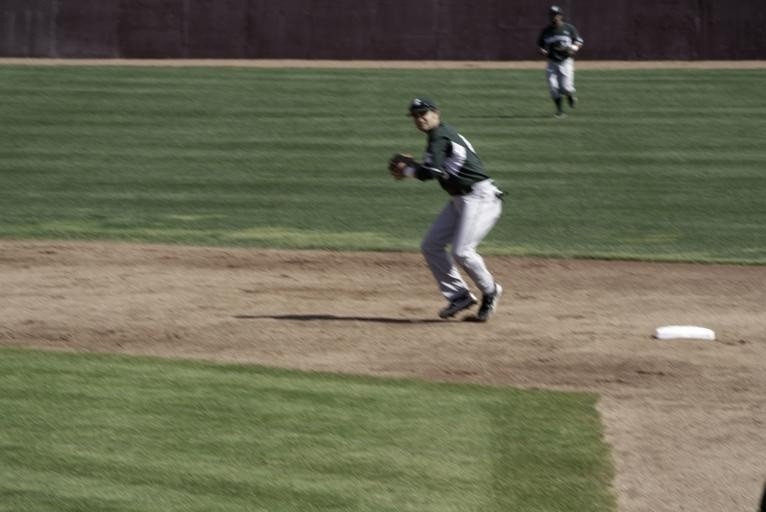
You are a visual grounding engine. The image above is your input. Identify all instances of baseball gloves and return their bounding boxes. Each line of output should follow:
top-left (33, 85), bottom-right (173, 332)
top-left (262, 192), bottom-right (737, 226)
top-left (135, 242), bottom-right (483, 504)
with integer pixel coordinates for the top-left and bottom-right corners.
top-left (548, 46), bottom-right (569, 62)
top-left (389, 153), bottom-right (413, 179)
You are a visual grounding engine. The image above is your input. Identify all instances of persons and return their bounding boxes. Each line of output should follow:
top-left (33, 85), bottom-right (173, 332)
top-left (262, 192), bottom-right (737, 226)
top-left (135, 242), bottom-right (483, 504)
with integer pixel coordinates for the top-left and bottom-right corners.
top-left (386, 96), bottom-right (508, 323)
top-left (535, 3), bottom-right (584, 118)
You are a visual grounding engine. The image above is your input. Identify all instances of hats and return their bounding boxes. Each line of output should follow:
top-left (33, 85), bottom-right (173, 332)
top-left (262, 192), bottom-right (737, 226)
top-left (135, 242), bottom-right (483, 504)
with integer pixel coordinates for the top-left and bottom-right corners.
top-left (407, 96), bottom-right (437, 117)
top-left (549, 5), bottom-right (564, 15)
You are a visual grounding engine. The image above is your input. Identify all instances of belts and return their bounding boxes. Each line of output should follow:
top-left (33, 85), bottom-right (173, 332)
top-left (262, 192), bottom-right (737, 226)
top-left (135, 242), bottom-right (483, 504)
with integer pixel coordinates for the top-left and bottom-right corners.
top-left (450, 187), bottom-right (472, 196)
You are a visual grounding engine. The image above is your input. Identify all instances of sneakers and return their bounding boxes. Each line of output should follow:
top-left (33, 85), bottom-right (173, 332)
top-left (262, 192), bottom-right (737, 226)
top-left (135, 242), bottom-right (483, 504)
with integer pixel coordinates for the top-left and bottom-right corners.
top-left (568, 95), bottom-right (575, 107)
top-left (479, 283), bottom-right (502, 321)
top-left (555, 109), bottom-right (564, 117)
top-left (440, 292), bottom-right (477, 318)
